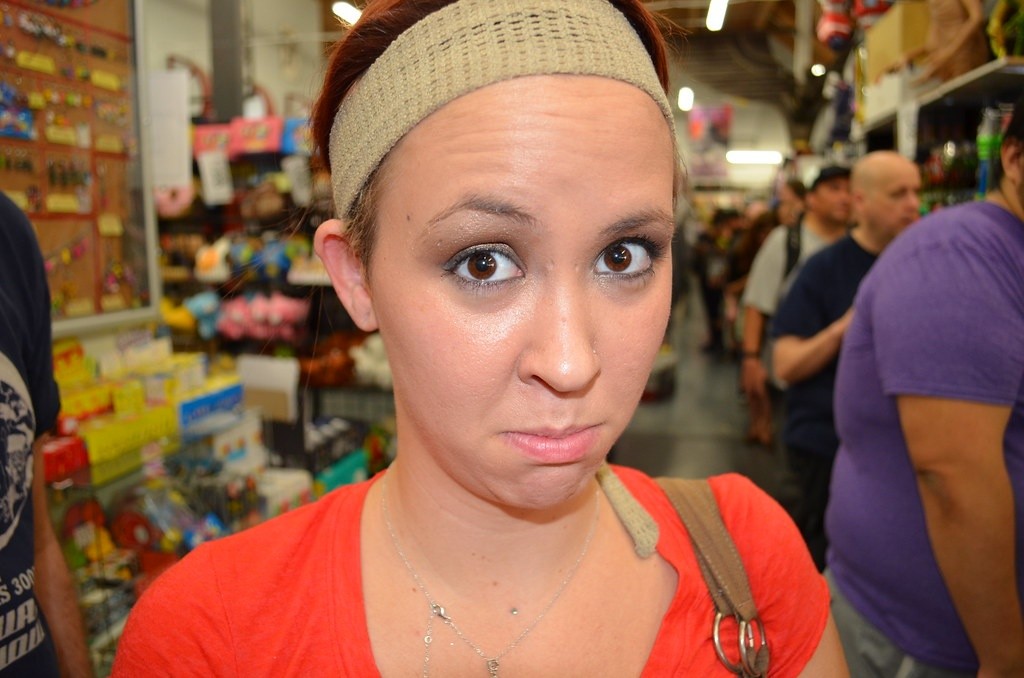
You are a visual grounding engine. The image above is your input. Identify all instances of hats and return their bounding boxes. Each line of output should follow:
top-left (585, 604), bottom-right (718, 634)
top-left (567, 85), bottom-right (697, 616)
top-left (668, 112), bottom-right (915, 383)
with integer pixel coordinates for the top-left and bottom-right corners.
top-left (799, 161), bottom-right (850, 193)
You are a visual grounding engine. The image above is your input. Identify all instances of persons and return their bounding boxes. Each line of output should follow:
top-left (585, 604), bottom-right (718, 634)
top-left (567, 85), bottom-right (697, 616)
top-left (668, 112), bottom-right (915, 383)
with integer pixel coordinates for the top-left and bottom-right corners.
top-left (763, 146), bottom-right (931, 588)
top-left (738, 163), bottom-right (857, 516)
top-left (0, 188), bottom-right (100, 678)
top-left (663, 173), bottom-right (807, 449)
top-left (97, 0), bottom-right (853, 678)
top-left (806, 87), bottom-right (1024, 678)
